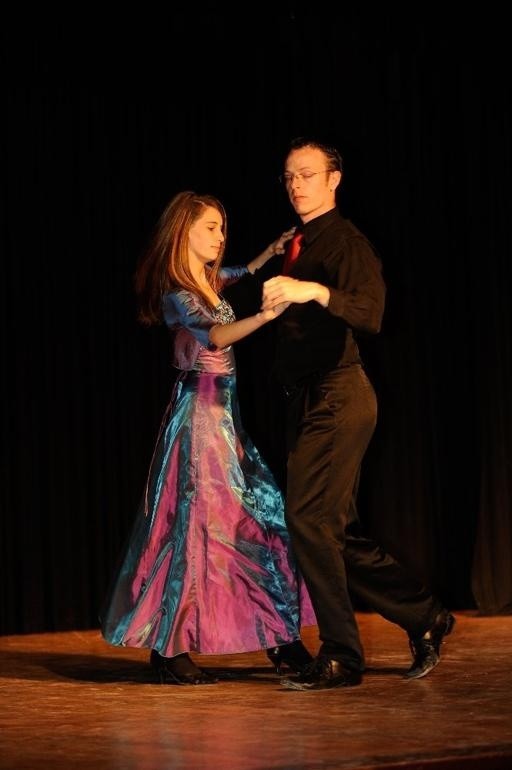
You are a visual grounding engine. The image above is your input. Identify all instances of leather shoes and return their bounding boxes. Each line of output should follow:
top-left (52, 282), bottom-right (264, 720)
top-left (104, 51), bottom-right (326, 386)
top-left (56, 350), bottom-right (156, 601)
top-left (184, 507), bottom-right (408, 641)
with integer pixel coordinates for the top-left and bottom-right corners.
top-left (402, 606), bottom-right (458, 681)
top-left (277, 653), bottom-right (366, 692)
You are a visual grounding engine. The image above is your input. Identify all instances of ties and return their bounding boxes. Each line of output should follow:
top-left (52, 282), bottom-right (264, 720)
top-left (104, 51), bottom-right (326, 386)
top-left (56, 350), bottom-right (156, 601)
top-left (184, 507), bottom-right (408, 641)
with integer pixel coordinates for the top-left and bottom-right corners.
top-left (282, 233), bottom-right (305, 275)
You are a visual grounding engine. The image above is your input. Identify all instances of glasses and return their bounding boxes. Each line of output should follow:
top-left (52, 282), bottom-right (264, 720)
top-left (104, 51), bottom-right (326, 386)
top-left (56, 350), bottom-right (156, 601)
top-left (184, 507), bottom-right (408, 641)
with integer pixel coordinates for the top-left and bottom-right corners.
top-left (279, 168), bottom-right (332, 187)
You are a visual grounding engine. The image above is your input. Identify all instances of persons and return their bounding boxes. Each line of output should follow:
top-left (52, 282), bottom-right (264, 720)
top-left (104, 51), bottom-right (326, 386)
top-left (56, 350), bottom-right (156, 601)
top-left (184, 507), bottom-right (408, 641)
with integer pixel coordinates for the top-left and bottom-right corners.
top-left (101, 190), bottom-right (319, 686)
top-left (261, 136), bottom-right (454, 692)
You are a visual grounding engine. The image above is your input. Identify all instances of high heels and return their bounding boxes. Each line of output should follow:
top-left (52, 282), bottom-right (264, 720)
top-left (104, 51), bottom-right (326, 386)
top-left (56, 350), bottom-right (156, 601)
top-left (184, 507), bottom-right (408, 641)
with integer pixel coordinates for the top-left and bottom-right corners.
top-left (150, 649), bottom-right (220, 686)
top-left (266, 640), bottom-right (316, 674)
